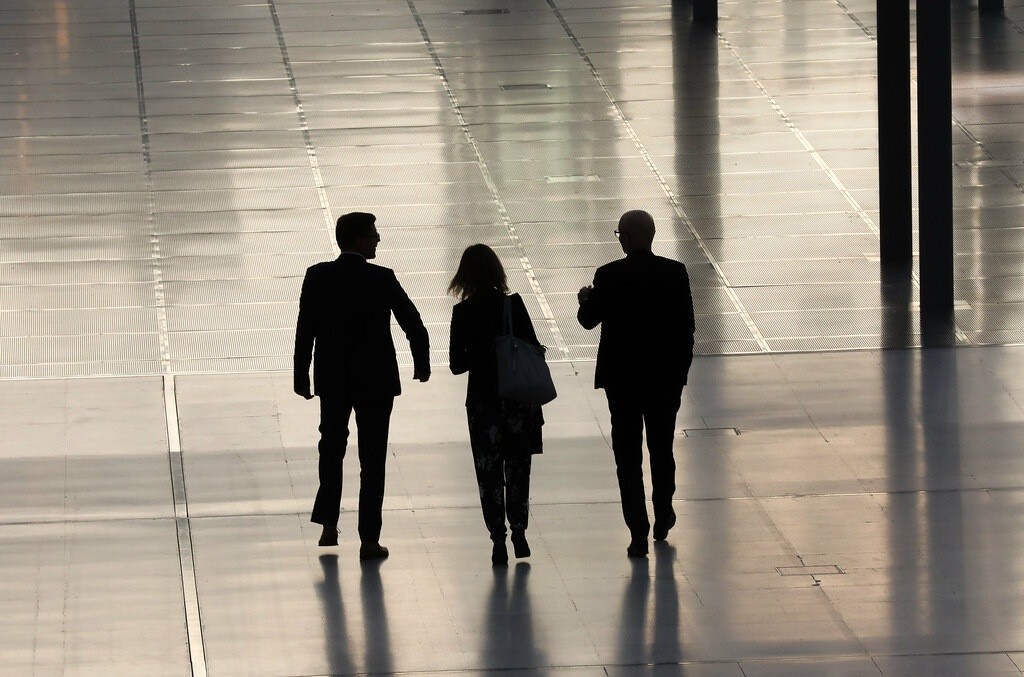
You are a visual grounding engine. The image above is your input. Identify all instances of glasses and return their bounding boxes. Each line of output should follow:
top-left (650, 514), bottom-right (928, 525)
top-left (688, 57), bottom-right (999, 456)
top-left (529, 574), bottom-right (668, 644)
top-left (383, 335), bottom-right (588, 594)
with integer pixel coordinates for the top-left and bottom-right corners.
top-left (614, 229), bottom-right (621, 238)
top-left (357, 233), bottom-right (379, 240)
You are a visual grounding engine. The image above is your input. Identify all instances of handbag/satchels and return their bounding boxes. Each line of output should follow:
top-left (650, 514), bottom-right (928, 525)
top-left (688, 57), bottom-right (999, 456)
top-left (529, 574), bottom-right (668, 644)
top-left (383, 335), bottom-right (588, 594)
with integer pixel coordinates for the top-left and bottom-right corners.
top-left (494, 296), bottom-right (557, 406)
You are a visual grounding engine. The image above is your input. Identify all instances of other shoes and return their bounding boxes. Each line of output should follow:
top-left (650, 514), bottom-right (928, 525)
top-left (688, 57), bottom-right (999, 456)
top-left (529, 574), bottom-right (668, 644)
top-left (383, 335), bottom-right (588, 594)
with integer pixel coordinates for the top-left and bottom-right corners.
top-left (652, 510), bottom-right (676, 540)
top-left (360, 543), bottom-right (389, 560)
top-left (511, 530), bottom-right (530, 558)
top-left (492, 547), bottom-right (508, 565)
top-left (627, 539), bottom-right (648, 555)
top-left (318, 525), bottom-right (341, 546)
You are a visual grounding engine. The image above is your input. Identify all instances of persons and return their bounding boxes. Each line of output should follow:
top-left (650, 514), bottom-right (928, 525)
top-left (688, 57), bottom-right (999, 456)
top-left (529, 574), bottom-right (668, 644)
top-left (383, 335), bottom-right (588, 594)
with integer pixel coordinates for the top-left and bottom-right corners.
top-left (577, 209), bottom-right (696, 557)
top-left (447, 243), bottom-right (544, 565)
top-left (293, 212), bottom-right (430, 561)
